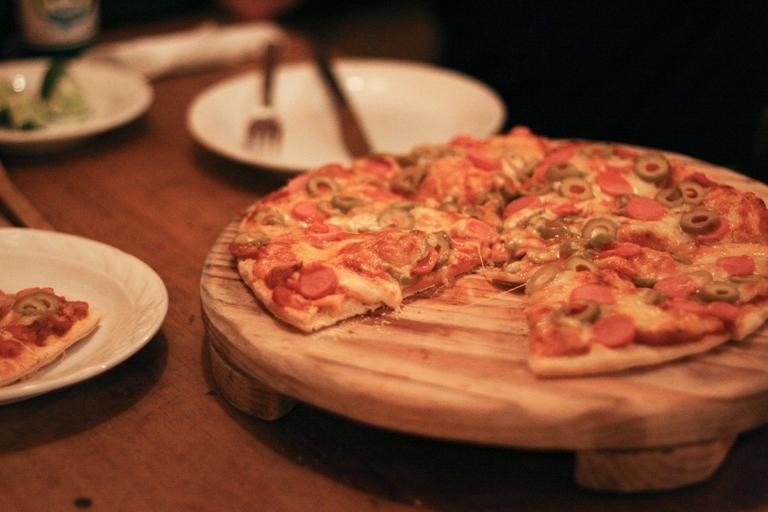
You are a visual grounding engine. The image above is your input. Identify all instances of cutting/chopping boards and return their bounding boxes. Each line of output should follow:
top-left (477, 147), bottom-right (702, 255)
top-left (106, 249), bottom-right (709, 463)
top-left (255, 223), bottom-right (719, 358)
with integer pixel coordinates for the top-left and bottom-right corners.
top-left (197, 138), bottom-right (768, 493)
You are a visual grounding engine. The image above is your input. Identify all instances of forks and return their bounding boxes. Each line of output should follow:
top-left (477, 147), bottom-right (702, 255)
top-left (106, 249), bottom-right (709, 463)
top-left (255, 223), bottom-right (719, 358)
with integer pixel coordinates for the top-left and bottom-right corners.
top-left (243, 43), bottom-right (283, 152)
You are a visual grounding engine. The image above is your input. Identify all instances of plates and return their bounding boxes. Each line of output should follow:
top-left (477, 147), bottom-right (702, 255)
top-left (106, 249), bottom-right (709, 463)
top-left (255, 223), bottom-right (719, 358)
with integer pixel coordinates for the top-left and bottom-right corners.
top-left (184, 56), bottom-right (507, 174)
top-left (0, 55), bottom-right (156, 157)
top-left (0, 227), bottom-right (169, 405)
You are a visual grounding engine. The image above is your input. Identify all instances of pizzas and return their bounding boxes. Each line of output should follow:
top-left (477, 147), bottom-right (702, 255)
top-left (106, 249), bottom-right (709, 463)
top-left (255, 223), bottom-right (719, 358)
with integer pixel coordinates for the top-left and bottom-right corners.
top-left (227, 125), bottom-right (764, 383)
top-left (1, 285), bottom-right (102, 388)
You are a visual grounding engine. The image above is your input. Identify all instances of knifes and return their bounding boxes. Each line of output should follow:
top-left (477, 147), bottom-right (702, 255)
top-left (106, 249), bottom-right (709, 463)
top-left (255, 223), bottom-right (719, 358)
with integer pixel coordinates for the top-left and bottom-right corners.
top-left (311, 50), bottom-right (375, 160)
